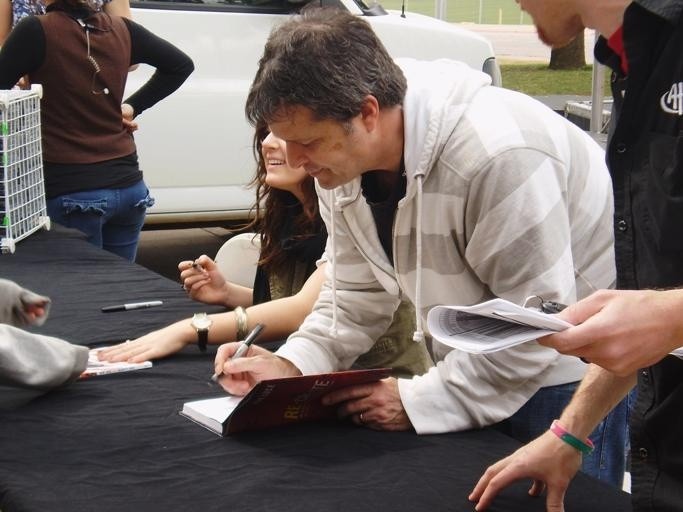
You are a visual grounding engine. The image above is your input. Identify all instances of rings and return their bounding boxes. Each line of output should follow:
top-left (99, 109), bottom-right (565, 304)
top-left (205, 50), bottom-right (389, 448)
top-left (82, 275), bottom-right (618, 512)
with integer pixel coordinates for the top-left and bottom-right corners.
top-left (358, 414), bottom-right (366, 423)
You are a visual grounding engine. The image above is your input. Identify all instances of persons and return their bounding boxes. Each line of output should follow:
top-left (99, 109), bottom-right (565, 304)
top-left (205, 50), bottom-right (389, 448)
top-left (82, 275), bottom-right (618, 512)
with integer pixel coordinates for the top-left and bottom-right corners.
top-left (1, 0), bottom-right (143, 93)
top-left (0, 275), bottom-right (91, 394)
top-left (1, 0), bottom-right (196, 267)
top-left (468, 2), bottom-right (683, 508)
top-left (210, 2), bottom-right (630, 491)
top-left (92, 109), bottom-right (435, 382)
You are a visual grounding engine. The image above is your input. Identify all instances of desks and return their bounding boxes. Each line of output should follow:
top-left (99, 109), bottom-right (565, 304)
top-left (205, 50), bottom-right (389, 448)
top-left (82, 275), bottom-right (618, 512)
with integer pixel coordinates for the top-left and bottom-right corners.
top-left (0, 228), bottom-right (683, 512)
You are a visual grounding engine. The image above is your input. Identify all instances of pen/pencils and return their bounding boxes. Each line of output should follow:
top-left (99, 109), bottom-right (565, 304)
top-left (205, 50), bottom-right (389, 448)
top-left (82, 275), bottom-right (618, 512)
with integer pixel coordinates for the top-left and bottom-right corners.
top-left (193, 263), bottom-right (204, 271)
top-left (101, 301), bottom-right (163, 313)
top-left (207, 324), bottom-right (263, 387)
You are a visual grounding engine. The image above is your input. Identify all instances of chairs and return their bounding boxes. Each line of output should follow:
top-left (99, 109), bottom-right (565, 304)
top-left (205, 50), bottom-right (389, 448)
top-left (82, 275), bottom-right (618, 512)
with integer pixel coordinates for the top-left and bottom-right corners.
top-left (214, 232), bottom-right (260, 290)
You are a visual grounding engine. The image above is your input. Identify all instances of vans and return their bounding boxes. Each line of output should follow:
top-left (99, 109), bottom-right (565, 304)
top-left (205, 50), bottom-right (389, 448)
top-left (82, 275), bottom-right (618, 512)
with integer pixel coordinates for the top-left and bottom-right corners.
top-left (121, 0), bottom-right (502, 231)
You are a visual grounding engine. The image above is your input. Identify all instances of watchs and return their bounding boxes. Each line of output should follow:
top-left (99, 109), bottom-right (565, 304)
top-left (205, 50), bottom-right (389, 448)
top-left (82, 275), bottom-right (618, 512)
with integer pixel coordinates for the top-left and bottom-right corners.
top-left (190, 310), bottom-right (212, 354)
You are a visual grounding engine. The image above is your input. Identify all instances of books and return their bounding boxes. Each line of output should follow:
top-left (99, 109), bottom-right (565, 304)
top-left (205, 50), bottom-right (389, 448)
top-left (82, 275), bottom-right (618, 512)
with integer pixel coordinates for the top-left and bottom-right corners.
top-left (72, 337), bottom-right (153, 383)
top-left (175, 365), bottom-right (394, 438)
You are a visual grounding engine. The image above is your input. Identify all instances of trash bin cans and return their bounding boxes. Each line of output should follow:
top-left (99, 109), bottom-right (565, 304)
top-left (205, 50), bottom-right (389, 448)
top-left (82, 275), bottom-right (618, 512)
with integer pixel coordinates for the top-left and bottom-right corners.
top-left (540, 300), bottom-right (569, 314)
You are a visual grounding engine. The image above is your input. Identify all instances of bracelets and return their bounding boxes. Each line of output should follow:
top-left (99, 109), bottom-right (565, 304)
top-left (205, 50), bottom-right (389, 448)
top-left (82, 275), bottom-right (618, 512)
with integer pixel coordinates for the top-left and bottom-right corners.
top-left (232, 304), bottom-right (248, 342)
top-left (548, 421), bottom-right (596, 457)
